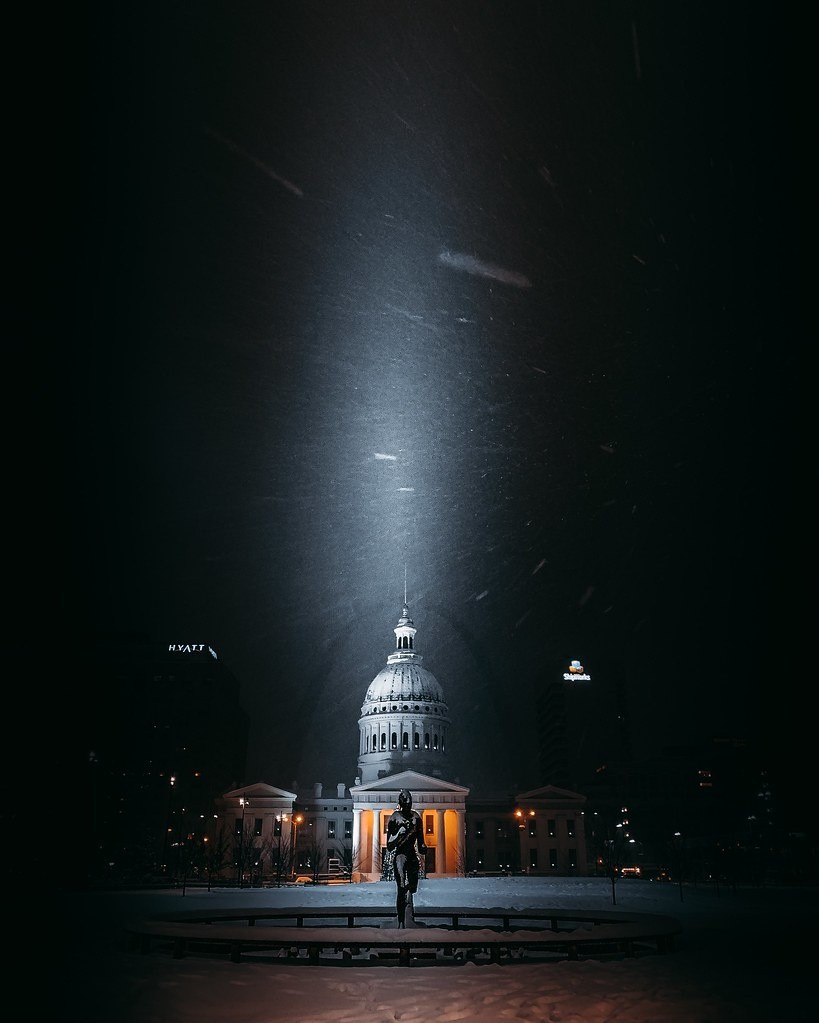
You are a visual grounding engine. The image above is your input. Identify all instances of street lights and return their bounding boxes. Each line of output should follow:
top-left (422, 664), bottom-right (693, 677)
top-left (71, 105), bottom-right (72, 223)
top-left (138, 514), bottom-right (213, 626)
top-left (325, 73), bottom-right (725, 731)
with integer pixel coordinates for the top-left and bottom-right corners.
top-left (282, 815), bottom-right (303, 876)
